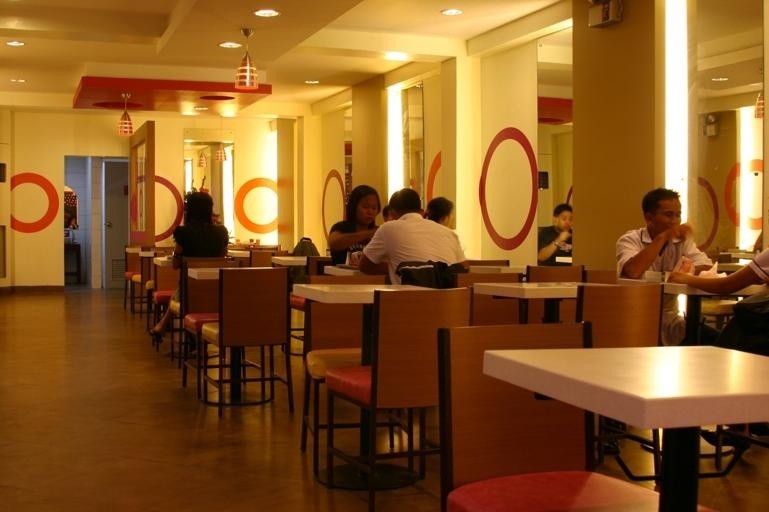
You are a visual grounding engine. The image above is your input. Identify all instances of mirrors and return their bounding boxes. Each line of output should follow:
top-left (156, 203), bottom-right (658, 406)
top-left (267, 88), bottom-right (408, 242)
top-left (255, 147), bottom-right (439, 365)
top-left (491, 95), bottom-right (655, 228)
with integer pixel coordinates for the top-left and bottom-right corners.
top-left (184, 128), bottom-right (236, 245)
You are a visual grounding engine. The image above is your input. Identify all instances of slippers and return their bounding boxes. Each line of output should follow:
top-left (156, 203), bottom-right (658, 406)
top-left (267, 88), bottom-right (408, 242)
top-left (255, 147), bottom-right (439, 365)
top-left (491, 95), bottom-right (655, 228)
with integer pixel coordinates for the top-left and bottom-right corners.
top-left (148, 328), bottom-right (164, 343)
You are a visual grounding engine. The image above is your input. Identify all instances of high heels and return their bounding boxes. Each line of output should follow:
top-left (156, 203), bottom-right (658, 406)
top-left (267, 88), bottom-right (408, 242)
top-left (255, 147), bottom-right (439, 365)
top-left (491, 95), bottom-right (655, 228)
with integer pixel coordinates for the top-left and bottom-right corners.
top-left (701, 429), bottom-right (752, 455)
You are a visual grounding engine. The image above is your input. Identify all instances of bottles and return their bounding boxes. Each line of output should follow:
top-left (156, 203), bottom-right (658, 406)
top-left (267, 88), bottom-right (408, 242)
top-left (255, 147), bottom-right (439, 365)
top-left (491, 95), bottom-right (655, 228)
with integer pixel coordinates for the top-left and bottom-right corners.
top-left (249, 238), bottom-right (260, 246)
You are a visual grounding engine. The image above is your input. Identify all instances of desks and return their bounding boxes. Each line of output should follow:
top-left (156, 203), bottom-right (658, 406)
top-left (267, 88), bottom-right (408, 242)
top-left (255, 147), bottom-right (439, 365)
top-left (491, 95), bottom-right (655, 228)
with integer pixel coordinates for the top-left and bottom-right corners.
top-left (65, 240), bottom-right (80, 284)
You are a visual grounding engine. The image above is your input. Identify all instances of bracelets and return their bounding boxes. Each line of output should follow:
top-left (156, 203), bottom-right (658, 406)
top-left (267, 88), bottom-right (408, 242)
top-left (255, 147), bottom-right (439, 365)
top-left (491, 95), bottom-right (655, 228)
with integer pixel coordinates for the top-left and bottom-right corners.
top-left (551, 241), bottom-right (563, 249)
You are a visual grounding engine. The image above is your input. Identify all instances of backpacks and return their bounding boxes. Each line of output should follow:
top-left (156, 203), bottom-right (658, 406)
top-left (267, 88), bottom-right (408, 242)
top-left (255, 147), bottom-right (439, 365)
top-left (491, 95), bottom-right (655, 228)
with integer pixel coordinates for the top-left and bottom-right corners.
top-left (288, 237), bottom-right (325, 280)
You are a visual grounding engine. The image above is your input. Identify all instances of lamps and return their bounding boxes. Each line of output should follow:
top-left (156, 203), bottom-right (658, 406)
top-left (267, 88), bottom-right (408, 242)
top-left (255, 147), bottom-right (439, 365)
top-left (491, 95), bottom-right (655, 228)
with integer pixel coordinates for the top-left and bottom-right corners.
top-left (754, 67), bottom-right (764, 118)
top-left (215, 115), bottom-right (226, 161)
top-left (198, 150), bottom-right (207, 167)
top-left (118, 93), bottom-right (133, 137)
top-left (234, 27), bottom-right (259, 90)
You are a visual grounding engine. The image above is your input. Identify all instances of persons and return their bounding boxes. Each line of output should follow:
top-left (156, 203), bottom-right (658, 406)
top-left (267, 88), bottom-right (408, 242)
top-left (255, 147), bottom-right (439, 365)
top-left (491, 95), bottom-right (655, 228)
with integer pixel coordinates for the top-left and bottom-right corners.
top-left (661, 246), bottom-right (769, 457)
top-left (383, 205), bottom-right (393, 221)
top-left (356, 187), bottom-right (470, 286)
top-left (147, 191), bottom-right (231, 352)
top-left (327, 184), bottom-right (383, 266)
top-left (422, 194), bottom-right (454, 229)
top-left (538, 202), bottom-right (573, 266)
top-left (612, 186), bottom-right (719, 346)
